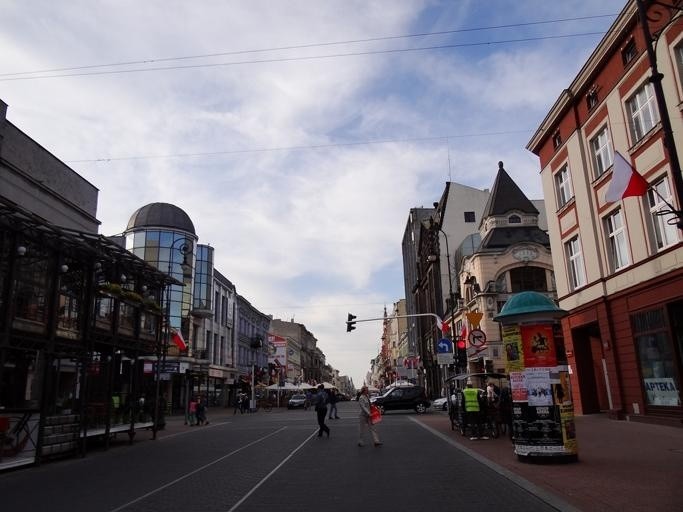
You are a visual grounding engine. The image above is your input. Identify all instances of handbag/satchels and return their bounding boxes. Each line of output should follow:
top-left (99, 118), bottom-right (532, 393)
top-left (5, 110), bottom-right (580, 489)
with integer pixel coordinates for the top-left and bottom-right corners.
top-left (370, 404), bottom-right (382, 424)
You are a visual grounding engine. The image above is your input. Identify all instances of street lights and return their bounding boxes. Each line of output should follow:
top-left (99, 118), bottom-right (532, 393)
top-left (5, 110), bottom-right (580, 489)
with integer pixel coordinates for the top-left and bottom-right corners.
top-left (423, 214), bottom-right (460, 388)
top-left (161, 237), bottom-right (194, 371)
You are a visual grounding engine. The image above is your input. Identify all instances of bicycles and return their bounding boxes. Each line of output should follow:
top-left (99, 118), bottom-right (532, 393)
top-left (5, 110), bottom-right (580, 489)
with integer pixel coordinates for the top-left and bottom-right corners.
top-left (255, 399), bottom-right (273, 413)
top-left (1, 400), bottom-right (87, 453)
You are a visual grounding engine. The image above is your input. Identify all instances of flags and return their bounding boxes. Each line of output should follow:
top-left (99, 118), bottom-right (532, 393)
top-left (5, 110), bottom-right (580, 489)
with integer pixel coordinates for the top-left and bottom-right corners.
top-left (461, 320), bottom-right (468, 340)
top-left (434, 316), bottom-right (449, 336)
top-left (171, 329), bottom-right (186, 352)
top-left (604, 149), bottom-right (648, 208)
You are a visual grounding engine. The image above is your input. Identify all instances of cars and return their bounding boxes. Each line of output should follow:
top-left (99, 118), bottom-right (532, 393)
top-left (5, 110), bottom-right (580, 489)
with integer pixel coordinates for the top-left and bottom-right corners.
top-left (433, 388), bottom-right (498, 410)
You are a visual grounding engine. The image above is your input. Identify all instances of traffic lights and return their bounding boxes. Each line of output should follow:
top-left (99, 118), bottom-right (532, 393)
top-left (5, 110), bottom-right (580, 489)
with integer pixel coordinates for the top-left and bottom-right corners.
top-left (457, 340), bottom-right (467, 367)
top-left (348, 314), bottom-right (356, 332)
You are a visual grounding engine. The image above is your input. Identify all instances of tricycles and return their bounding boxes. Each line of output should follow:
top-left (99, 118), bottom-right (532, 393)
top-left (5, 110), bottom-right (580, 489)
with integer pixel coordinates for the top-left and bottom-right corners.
top-left (445, 372), bottom-right (514, 438)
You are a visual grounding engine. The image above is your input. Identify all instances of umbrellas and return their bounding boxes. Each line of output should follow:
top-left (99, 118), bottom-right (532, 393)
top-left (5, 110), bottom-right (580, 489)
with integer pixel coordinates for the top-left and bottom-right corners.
top-left (297, 382), bottom-right (315, 389)
top-left (267, 382), bottom-right (298, 391)
top-left (313, 381), bottom-right (336, 389)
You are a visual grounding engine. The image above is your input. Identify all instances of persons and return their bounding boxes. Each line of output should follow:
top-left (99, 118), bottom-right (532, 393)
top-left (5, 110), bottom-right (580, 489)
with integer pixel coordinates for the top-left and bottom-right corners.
top-left (110, 388), bottom-right (167, 431)
top-left (357, 386), bottom-right (383, 448)
top-left (554, 383), bottom-right (565, 404)
top-left (311, 384), bottom-right (329, 436)
top-left (535, 333), bottom-right (546, 347)
top-left (187, 396), bottom-right (197, 425)
top-left (306, 389), bottom-right (312, 409)
top-left (232, 392), bottom-right (243, 415)
top-left (530, 335), bottom-right (538, 349)
top-left (194, 402), bottom-right (207, 426)
top-left (505, 343), bottom-right (518, 361)
top-left (199, 399), bottom-right (209, 425)
top-left (242, 392), bottom-right (249, 414)
top-left (327, 387), bottom-right (339, 420)
top-left (460, 378), bottom-right (490, 441)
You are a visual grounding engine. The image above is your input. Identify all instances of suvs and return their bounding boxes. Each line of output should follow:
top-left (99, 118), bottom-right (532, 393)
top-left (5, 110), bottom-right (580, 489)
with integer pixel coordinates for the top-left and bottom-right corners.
top-left (370, 385), bottom-right (431, 415)
top-left (289, 394), bottom-right (307, 409)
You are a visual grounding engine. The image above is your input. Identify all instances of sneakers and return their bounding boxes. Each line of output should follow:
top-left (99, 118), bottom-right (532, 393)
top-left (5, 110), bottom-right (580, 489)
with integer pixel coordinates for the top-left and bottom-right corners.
top-left (469, 436), bottom-right (489, 441)
